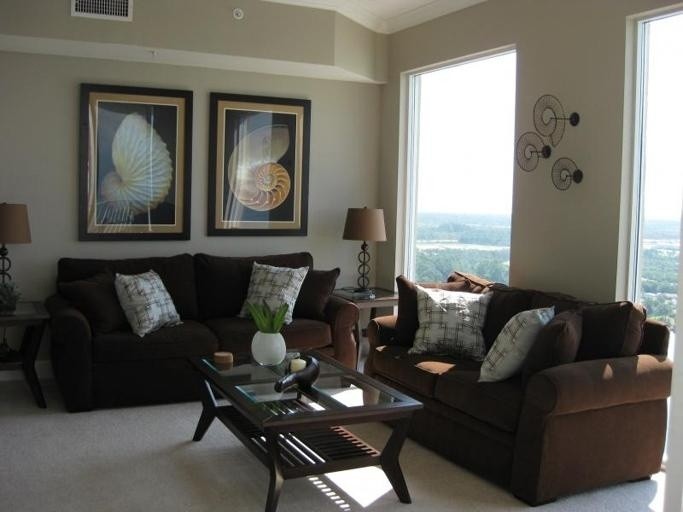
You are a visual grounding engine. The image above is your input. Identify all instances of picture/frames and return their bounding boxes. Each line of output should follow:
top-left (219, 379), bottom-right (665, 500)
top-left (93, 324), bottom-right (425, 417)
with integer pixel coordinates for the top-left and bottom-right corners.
top-left (78, 82), bottom-right (195, 242)
top-left (207, 91), bottom-right (312, 238)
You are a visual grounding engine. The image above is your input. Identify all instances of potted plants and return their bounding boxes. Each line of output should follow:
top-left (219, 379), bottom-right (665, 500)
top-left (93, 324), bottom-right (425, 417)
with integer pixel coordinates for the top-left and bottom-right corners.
top-left (247, 299), bottom-right (290, 366)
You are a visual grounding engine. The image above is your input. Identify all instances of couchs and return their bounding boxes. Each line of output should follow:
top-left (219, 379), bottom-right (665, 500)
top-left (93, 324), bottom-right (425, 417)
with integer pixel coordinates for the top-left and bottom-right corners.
top-left (365, 273), bottom-right (676, 506)
top-left (44, 251), bottom-right (360, 412)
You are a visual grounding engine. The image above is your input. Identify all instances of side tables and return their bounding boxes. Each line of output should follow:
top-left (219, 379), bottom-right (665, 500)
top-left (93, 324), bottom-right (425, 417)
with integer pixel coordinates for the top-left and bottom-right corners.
top-left (334, 284), bottom-right (399, 359)
top-left (0, 299), bottom-right (50, 409)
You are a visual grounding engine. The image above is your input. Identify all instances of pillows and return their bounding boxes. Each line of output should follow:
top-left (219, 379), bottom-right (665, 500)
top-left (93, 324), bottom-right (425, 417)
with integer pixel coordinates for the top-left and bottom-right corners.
top-left (238, 260), bottom-right (310, 324)
top-left (397, 275), bottom-right (583, 396)
top-left (115, 269), bottom-right (183, 337)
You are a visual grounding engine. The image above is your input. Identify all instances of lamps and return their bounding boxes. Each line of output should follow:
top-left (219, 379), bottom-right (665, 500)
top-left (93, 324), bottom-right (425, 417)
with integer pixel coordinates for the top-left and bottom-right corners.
top-left (0, 202), bottom-right (33, 310)
top-left (341, 207), bottom-right (387, 294)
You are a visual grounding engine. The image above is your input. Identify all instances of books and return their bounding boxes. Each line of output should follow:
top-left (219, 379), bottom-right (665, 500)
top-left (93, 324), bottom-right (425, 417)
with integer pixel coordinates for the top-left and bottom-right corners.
top-left (202, 364), bottom-right (299, 410)
